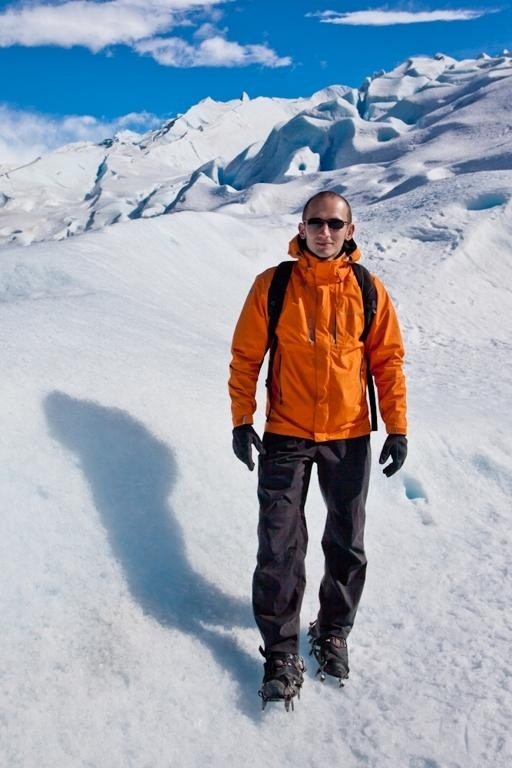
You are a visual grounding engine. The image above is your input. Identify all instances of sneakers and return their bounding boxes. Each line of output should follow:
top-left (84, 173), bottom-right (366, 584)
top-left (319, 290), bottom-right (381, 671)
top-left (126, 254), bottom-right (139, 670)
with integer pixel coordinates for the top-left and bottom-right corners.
top-left (310, 619), bottom-right (349, 679)
top-left (263, 652), bottom-right (304, 700)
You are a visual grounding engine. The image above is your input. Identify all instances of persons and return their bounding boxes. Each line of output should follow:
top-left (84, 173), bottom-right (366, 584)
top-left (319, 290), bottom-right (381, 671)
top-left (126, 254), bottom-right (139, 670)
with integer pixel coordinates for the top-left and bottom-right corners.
top-left (227, 191), bottom-right (408, 699)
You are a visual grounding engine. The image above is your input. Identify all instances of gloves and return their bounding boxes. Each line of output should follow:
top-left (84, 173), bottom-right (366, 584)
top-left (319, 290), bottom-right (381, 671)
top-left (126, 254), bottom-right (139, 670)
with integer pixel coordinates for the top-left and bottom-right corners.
top-left (379, 435), bottom-right (407, 477)
top-left (233, 424), bottom-right (267, 472)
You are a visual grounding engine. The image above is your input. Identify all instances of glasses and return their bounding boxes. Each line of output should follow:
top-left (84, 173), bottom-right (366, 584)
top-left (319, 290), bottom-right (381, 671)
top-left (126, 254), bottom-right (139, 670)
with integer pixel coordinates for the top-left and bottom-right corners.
top-left (302, 216), bottom-right (351, 230)
